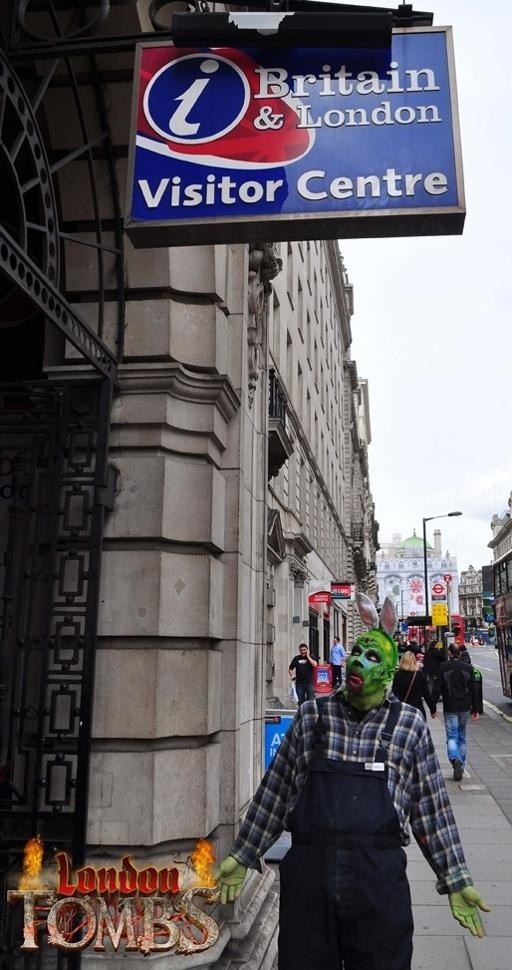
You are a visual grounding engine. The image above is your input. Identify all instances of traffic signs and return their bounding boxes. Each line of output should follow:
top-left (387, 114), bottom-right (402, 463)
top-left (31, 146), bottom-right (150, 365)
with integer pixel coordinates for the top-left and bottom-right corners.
top-left (431, 596), bottom-right (448, 627)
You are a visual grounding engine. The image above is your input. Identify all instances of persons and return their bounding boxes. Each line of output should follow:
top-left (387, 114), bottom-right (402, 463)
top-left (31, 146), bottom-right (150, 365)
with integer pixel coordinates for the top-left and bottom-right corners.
top-left (211, 632), bottom-right (490, 970)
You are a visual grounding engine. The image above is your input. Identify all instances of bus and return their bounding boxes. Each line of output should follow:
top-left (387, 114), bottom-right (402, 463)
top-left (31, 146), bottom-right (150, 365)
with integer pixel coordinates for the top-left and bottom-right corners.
top-left (407, 612), bottom-right (492, 650)
top-left (491, 546), bottom-right (512, 702)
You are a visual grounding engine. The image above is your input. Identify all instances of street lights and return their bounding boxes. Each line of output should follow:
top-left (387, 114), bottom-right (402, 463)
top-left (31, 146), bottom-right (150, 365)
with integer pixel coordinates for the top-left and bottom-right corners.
top-left (421, 508), bottom-right (463, 649)
top-left (395, 586), bottom-right (418, 621)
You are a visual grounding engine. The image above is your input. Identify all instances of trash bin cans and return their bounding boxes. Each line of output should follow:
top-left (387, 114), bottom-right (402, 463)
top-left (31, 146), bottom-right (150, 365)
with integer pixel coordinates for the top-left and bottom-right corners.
top-left (474, 668), bottom-right (483, 714)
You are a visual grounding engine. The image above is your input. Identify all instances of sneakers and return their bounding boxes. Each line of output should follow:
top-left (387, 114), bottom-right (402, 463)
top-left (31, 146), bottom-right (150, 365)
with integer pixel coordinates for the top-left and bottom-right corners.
top-left (453, 758), bottom-right (462, 779)
